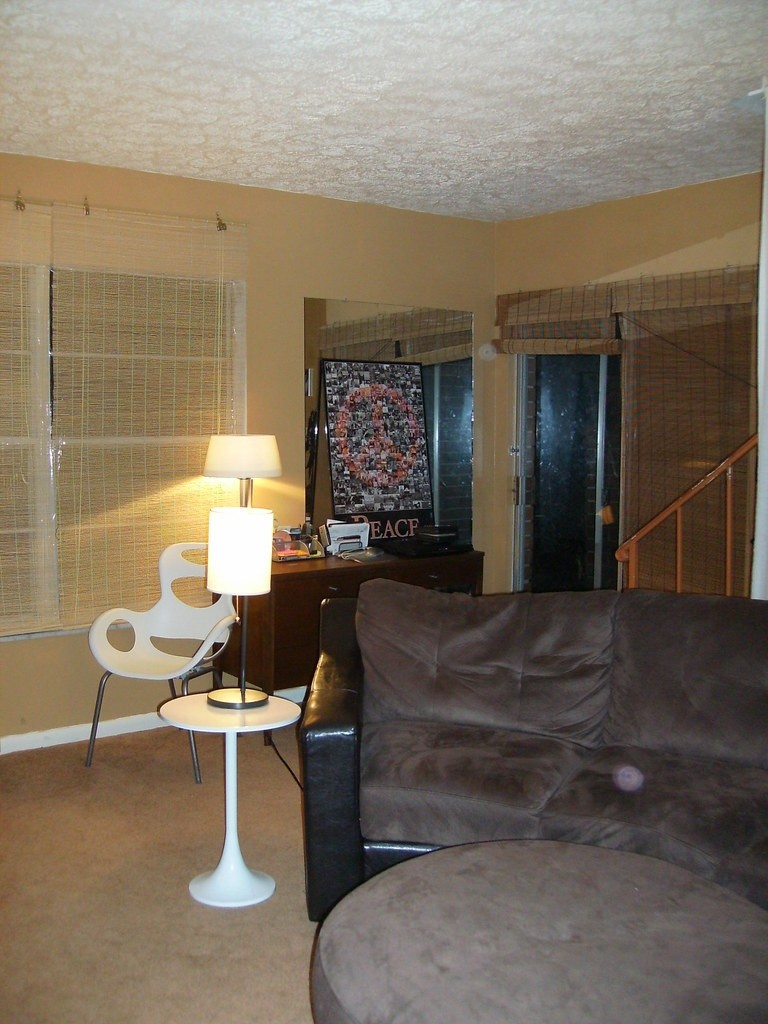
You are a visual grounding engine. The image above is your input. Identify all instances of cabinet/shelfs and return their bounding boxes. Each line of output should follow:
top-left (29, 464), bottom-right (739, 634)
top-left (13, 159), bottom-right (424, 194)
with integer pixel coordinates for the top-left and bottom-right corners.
top-left (211, 546), bottom-right (486, 746)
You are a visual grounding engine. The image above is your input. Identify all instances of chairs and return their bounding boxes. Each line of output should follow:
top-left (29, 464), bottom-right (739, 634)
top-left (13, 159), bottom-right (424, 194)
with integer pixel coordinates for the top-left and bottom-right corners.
top-left (85, 542), bottom-right (242, 785)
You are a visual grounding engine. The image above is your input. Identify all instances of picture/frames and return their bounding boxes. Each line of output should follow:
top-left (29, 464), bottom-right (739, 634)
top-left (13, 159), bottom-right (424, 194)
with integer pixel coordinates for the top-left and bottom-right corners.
top-left (321, 358), bottom-right (435, 545)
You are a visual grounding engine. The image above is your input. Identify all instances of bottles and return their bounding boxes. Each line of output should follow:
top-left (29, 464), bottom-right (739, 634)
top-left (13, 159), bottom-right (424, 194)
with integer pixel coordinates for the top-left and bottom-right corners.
top-left (302, 517), bottom-right (313, 535)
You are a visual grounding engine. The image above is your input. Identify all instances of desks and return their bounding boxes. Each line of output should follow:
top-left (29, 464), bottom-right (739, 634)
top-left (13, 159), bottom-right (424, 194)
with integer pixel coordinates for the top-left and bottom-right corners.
top-left (308, 839), bottom-right (768, 1024)
top-left (156, 693), bottom-right (302, 908)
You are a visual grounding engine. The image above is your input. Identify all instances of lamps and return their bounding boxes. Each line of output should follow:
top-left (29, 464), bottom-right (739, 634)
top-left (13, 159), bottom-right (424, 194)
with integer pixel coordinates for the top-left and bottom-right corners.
top-left (201, 432), bottom-right (283, 507)
top-left (205, 507), bottom-right (274, 709)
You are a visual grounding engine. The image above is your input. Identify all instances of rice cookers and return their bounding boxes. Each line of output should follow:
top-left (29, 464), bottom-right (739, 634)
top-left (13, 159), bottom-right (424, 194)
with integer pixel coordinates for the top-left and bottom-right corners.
top-left (409, 524), bottom-right (459, 556)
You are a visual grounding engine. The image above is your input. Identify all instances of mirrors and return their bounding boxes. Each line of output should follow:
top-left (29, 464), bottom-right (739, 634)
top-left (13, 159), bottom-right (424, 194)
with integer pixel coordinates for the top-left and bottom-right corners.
top-left (303, 296), bottom-right (475, 556)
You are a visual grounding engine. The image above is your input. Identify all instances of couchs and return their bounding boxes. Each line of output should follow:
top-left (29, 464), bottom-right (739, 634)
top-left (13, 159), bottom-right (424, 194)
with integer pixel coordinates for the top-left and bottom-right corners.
top-left (298, 577), bottom-right (768, 922)
